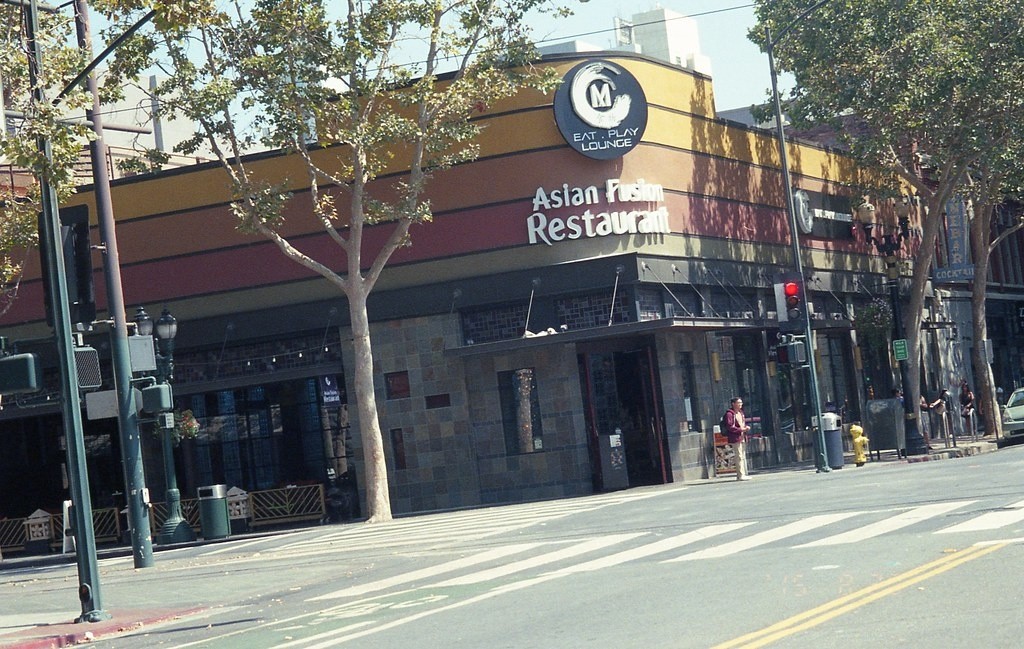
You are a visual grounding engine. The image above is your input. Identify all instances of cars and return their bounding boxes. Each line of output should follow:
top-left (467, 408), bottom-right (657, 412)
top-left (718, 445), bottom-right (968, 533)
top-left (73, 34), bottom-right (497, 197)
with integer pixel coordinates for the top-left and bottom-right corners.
top-left (1002, 388), bottom-right (1024, 439)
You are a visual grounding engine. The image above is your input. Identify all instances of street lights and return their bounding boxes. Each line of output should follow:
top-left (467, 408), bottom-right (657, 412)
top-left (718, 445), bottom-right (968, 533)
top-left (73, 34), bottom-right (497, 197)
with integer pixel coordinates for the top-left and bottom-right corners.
top-left (131, 305), bottom-right (196, 544)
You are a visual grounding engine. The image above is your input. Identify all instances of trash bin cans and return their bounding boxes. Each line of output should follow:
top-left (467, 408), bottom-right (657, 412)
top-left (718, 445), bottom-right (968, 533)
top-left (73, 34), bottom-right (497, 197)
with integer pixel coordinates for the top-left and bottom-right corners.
top-left (197, 484), bottom-right (231, 540)
top-left (811, 413), bottom-right (844, 470)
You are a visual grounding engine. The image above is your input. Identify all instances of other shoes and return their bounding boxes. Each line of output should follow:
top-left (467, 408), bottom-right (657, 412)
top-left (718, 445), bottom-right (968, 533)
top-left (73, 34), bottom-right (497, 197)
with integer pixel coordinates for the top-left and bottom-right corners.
top-left (737, 475), bottom-right (752, 481)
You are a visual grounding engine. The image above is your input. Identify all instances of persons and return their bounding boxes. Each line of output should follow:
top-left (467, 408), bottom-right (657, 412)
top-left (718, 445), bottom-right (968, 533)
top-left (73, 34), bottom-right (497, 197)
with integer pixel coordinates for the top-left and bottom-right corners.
top-left (892, 389), bottom-right (904, 407)
top-left (719, 397), bottom-right (752, 481)
top-left (959, 383), bottom-right (976, 434)
top-left (979, 387), bottom-right (984, 418)
top-left (930, 390), bottom-right (958, 439)
top-left (919, 394), bottom-right (933, 449)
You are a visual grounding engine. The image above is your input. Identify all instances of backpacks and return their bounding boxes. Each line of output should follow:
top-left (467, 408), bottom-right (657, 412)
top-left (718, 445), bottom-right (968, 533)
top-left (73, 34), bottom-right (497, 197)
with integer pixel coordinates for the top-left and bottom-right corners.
top-left (720, 411), bottom-right (729, 437)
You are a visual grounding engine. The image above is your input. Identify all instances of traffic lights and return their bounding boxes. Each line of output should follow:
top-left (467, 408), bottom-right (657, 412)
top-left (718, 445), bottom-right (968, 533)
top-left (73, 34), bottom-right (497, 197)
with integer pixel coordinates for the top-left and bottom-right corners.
top-left (774, 272), bottom-right (807, 333)
